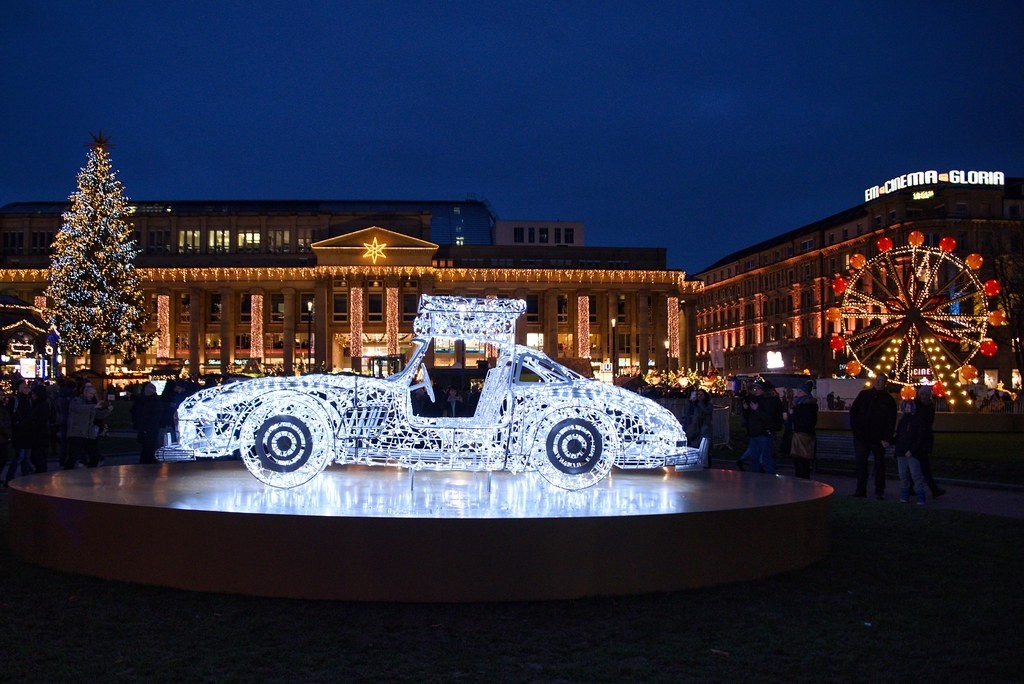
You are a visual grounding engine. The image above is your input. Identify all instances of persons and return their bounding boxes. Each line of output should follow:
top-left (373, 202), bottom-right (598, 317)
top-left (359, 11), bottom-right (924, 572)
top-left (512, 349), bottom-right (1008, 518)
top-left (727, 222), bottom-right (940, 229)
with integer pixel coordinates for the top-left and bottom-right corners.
top-left (409, 381), bottom-right (482, 417)
top-left (682, 371), bottom-right (945, 506)
top-left (0, 373), bottom-right (252, 488)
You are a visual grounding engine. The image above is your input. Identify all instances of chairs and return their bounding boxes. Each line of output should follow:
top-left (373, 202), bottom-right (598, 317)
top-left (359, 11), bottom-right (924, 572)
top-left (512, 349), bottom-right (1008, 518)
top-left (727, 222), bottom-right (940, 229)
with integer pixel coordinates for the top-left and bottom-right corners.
top-left (435, 367), bottom-right (512, 428)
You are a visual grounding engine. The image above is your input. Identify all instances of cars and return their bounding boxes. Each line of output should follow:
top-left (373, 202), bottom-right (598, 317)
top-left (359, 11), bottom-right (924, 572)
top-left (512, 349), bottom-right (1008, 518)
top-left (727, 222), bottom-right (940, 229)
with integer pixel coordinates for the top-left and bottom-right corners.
top-left (974, 383), bottom-right (1017, 405)
top-left (154, 294), bottom-right (711, 489)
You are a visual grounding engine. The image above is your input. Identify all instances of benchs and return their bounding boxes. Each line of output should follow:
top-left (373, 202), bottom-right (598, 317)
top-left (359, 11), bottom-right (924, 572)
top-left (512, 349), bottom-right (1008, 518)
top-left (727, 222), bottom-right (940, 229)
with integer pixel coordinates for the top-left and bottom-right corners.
top-left (812, 434), bottom-right (896, 476)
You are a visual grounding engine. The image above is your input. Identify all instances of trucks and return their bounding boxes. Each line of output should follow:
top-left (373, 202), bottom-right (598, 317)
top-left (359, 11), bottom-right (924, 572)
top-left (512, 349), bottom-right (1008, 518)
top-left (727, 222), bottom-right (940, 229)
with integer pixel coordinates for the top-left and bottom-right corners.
top-left (734, 371), bottom-right (877, 411)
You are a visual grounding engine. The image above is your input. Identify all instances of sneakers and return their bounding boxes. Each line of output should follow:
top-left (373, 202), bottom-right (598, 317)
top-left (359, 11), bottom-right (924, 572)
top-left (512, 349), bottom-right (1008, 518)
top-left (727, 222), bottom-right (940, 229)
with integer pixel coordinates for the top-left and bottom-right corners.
top-left (930, 483), bottom-right (945, 498)
top-left (917, 490), bottom-right (926, 506)
top-left (899, 489), bottom-right (908, 503)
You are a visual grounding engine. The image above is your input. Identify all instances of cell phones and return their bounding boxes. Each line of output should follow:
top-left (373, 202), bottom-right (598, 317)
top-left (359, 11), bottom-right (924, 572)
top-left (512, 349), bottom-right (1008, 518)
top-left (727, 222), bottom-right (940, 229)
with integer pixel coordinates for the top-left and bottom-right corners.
top-left (108, 394), bottom-right (116, 400)
top-left (691, 390), bottom-right (697, 401)
top-left (120, 391), bottom-right (127, 397)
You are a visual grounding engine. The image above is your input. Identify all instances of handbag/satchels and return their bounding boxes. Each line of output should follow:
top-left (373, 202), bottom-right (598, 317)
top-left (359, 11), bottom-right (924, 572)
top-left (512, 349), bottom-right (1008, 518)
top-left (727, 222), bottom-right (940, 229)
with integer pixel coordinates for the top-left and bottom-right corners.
top-left (789, 431), bottom-right (816, 462)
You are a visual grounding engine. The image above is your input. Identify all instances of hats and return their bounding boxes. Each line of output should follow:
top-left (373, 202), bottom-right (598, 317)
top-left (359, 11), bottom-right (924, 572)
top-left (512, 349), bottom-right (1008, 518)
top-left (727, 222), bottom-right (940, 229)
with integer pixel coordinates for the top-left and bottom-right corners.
top-left (175, 380), bottom-right (186, 389)
top-left (755, 379), bottom-right (772, 390)
top-left (919, 384), bottom-right (932, 393)
top-left (798, 381), bottom-right (814, 394)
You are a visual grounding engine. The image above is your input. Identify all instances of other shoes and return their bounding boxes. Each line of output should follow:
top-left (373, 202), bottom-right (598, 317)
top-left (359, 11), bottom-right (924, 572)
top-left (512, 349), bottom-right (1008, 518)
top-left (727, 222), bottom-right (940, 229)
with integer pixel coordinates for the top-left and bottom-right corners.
top-left (850, 490), bottom-right (866, 499)
top-left (874, 489), bottom-right (886, 499)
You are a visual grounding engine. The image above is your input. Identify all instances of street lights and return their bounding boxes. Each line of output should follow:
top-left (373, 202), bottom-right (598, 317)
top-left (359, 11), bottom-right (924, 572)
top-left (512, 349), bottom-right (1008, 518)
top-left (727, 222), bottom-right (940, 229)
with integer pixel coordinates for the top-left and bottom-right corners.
top-left (665, 340), bottom-right (670, 398)
top-left (611, 317), bottom-right (617, 386)
top-left (306, 300), bottom-right (313, 374)
